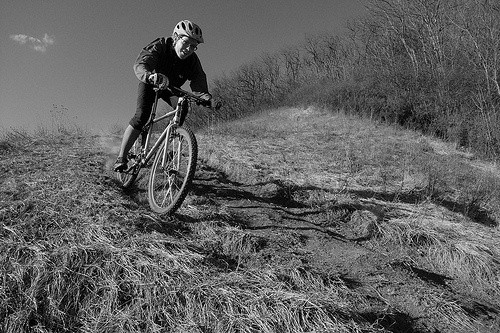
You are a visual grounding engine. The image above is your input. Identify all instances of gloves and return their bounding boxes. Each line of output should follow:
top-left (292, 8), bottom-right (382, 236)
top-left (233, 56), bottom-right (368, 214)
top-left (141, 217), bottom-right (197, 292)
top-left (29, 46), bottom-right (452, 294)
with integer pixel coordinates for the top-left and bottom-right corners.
top-left (148, 72), bottom-right (169, 91)
top-left (196, 92), bottom-right (212, 107)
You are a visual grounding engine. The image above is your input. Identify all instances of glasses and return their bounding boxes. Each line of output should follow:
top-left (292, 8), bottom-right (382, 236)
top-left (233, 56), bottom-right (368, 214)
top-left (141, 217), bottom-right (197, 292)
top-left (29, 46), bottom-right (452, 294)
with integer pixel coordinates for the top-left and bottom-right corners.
top-left (179, 36), bottom-right (198, 51)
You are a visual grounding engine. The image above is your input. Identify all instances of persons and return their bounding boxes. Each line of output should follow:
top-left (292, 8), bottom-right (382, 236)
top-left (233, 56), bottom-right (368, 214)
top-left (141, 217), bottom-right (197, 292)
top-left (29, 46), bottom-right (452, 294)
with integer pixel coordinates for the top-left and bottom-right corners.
top-left (112, 20), bottom-right (211, 173)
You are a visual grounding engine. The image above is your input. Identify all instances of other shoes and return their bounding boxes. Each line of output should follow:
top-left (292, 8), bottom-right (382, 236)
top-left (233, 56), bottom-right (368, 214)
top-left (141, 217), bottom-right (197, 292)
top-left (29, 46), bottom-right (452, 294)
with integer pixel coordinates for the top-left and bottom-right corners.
top-left (113, 155), bottom-right (128, 172)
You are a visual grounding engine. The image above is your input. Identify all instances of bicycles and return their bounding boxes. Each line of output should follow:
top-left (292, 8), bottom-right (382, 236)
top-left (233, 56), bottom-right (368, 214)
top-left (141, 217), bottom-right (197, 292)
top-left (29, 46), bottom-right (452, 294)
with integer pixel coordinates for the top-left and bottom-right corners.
top-left (116, 85), bottom-right (223, 215)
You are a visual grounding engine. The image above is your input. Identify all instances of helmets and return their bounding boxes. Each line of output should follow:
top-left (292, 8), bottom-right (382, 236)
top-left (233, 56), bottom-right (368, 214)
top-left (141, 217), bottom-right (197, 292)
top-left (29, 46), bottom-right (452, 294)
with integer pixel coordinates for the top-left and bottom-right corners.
top-left (173, 19), bottom-right (204, 43)
